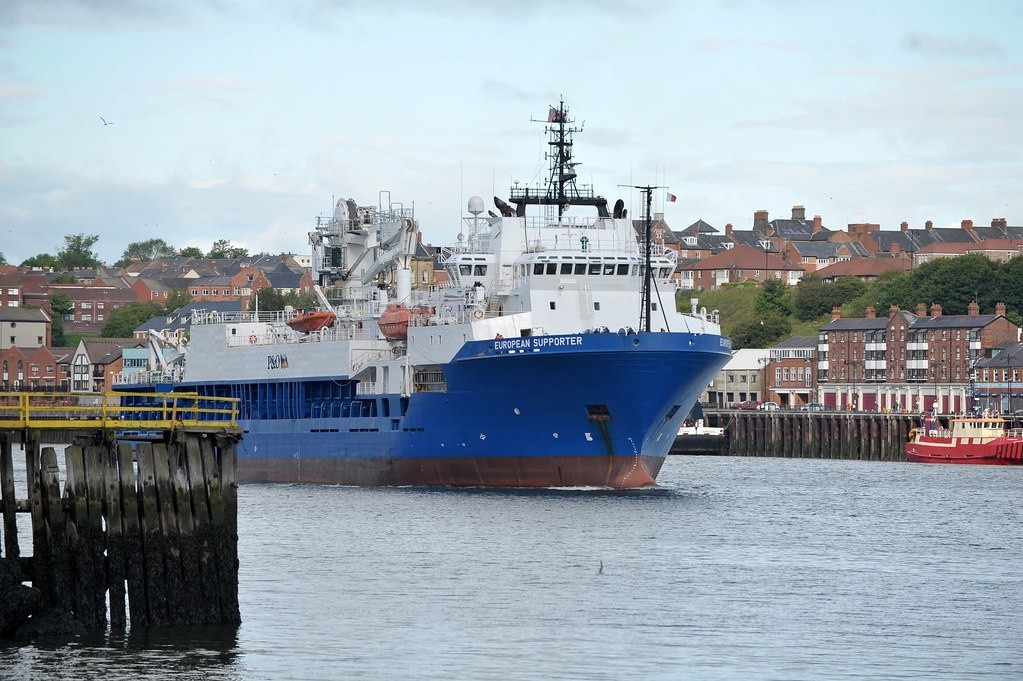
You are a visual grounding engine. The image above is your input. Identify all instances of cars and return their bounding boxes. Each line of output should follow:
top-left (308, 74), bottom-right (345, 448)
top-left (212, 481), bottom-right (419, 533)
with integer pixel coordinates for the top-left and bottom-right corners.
top-left (799, 401), bottom-right (824, 411)
top-left (755, 401), bottom-right (780, 412)
top-left (737, 400), bottom-right (760, 410)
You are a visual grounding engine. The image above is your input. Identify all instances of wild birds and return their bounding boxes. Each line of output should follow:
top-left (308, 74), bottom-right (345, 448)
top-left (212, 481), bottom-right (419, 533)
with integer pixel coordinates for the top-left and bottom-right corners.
top-left (100, 116), bottom-right (115, 126)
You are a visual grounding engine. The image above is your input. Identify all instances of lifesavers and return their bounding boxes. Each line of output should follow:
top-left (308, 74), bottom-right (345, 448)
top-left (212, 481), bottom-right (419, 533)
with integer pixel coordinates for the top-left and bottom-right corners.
top-left (250, 334), bottom-right (258, 344)
top-left (473, 309), bottom-right (484, 320)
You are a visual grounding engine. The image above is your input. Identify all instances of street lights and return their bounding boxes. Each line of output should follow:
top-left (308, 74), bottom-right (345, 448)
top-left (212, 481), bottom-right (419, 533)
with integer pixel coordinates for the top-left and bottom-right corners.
top-left (757, 358), bottom-right (772, 411)
top-left (806, 358), bottom-right (820, 412)
top-left (930, 362), bottom-right (945, 400)
top-left (1000, 356), bottom-right (1018, 414)
top-left (842, 360), bottom-right (864, 413)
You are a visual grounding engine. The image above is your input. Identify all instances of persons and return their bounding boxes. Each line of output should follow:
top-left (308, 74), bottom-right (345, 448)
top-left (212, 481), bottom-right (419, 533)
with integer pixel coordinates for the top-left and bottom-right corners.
top-left (364, 211), bottom-right (370, 224)
top-left (949, 410), bottom-right (1015, 430)
top-left (913, 401), bottom-right (918, 414)
top-left (893, 400), bottom-right (898, 412)
top-left (872, 401), bottom-right (878, 413)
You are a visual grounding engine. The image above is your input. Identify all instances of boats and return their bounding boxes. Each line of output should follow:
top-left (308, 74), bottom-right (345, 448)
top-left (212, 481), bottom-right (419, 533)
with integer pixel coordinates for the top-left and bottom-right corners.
top-left (668, 400), bottom-right (730, 457)
top-left (285, 311), bottom-right (336, 333)
top-left (904, 398), bottom-right (1022, 466)
top-left (110, 92), bottom-right (734, 489)
top-left (376, 303), bottom-right (430, 340)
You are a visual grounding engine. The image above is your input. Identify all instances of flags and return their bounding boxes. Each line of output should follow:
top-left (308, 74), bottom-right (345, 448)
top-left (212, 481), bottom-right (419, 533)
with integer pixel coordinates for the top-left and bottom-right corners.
top-left (666, 192), bottom-right (677, 202)
